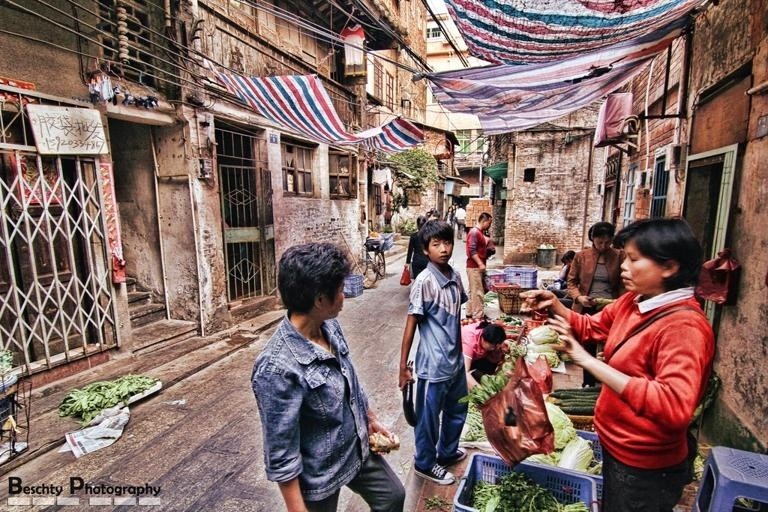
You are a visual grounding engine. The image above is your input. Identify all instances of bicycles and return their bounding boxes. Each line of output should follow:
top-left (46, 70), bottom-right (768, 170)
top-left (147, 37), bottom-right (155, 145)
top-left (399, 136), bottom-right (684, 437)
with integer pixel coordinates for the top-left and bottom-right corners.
top-left (348, 241), bottom-right (387, 291)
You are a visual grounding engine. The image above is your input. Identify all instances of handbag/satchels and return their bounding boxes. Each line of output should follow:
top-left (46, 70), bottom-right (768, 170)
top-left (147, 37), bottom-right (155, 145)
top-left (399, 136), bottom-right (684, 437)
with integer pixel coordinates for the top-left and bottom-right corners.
top-left (400, 268), bottom-right (411, 285)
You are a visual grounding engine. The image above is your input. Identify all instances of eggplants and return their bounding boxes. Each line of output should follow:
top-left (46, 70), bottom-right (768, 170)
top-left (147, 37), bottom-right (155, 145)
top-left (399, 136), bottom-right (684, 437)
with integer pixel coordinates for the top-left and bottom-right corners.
top-left (401, 360), bottom-right (417, 429)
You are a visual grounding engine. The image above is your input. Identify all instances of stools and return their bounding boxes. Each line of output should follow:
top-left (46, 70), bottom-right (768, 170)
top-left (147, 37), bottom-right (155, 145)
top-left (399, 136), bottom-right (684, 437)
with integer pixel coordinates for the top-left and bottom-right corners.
top-left (692, 444), bottom-right (767, 511)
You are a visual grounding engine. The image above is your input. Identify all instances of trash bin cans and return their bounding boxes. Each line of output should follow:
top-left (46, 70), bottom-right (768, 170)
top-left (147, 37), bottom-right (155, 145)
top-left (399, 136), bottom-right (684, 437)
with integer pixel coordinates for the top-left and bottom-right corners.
top-left (537, 245), bottom-right (558, 268)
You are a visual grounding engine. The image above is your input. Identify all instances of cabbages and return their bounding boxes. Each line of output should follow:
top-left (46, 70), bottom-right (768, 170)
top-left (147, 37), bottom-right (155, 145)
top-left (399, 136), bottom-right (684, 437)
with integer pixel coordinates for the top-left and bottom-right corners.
top-left (526, 326), bottom-right (558, 365)
top-left (526, 399), bottom-right (601, 474)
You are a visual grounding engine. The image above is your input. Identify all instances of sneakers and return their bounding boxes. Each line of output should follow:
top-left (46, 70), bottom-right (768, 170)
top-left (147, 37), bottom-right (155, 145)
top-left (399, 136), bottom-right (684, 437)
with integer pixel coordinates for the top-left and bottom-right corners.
top-left (414, 462), bottom-right (456, 485)
top-left (438, 448), bottom-right (466, 468)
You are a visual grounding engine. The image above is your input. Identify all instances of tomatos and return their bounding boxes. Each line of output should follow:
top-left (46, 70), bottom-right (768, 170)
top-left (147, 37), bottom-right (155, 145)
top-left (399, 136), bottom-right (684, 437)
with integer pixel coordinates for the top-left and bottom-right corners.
top-left (461, 319), bottom-right (481, 326)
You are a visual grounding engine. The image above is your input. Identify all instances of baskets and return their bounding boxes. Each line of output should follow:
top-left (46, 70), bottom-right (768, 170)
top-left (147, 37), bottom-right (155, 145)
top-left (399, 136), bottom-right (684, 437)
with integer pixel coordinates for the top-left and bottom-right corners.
top-left (452, 429), bottom-right (604, 512)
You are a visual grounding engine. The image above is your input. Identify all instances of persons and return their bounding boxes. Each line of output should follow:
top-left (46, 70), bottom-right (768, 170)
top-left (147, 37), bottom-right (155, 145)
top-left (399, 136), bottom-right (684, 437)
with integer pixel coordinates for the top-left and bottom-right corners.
top-left (398, 219), bottom-right (470, 486)
top-left (566, 222), bottom-right (623, 387)
top-left (251, 241), bottom-right (406, 511)
top-left (456, 323), bottom-right (508, 392)
top-left (558, 252), bottom-right (576, 289)
top-left (520, 216), bottom-right (717, 512)
top-left (402, 204), bottom-right (495, 320)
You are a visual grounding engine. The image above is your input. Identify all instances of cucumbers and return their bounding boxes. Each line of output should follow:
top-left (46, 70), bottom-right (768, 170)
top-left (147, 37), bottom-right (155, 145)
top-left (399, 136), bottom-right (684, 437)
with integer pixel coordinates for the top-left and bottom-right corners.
top-left (548, 388), bottom-right (599, 414)
